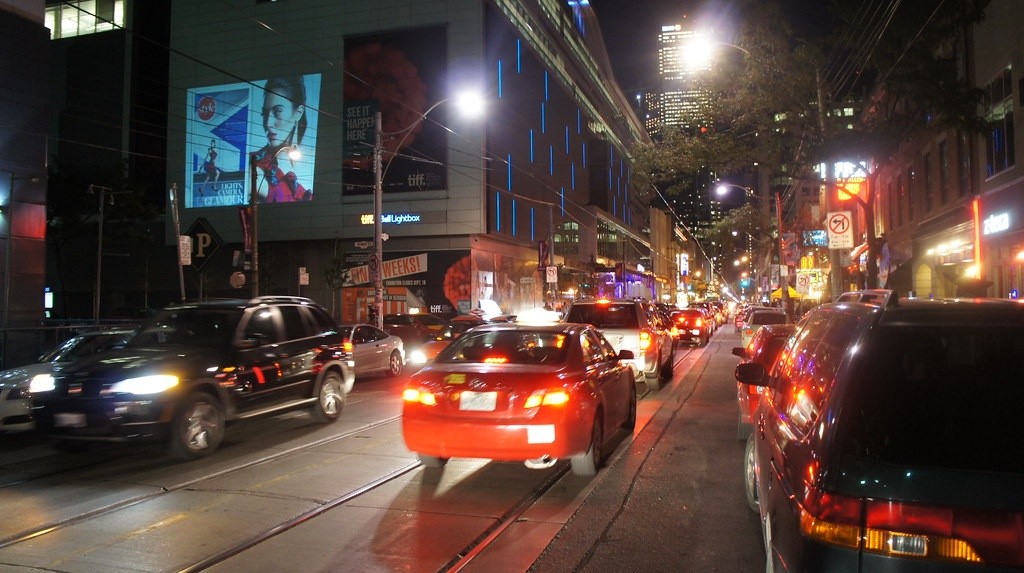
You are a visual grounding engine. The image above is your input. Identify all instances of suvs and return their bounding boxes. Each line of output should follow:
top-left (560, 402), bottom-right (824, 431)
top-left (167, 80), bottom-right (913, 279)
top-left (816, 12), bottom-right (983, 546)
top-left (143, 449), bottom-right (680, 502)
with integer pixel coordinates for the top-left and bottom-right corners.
top-left (28, 294), bottom-right (356, 460)
top-left (734, 289), bottom-right (1023, 573)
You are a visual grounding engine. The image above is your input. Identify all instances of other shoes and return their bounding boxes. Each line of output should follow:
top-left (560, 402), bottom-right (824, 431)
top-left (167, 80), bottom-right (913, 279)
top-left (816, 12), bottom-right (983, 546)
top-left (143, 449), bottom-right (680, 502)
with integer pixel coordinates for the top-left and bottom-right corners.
top-left (212, 185), bottom-right (218, 191)
top-left (199, 188), bottom-right (204, 197)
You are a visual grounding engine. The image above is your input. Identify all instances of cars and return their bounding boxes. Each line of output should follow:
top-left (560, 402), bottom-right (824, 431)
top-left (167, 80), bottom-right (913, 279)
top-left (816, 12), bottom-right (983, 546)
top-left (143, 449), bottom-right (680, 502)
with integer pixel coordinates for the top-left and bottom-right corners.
top-left (731, 322), bottom-right (802, 440)
top-left (654, 301), bottom-right (784, 336)
top-left (561, 297), bottom-right (680, 389)
top-left (336, 324), bottom-right (406, 377)
top-left (401, 323), bottom-right (637, 476)
top-left (669, 308), bottom-right (713, 347)
top-left (1, 328), bottom-right (179, 434)
top-left (740, 310), bottom-right (788, 353)
top-left (382, 312), bottom-right (520, 372)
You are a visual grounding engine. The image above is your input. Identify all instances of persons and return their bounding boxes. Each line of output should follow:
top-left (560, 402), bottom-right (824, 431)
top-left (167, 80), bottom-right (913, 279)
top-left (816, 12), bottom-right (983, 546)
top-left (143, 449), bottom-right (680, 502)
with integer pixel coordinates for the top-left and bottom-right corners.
top-left (247, 76), bottom-right (314, 203)
top-left (198, 138), bottom-right (220, 195)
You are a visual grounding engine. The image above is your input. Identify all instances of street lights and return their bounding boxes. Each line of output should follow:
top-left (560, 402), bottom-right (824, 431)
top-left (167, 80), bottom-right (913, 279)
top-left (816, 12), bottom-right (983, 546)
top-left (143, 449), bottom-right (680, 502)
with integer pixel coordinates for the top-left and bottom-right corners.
top-left (374, 93), bottom-right (488, 330)
top-left (679, 35), bottom-right (773, 280)
